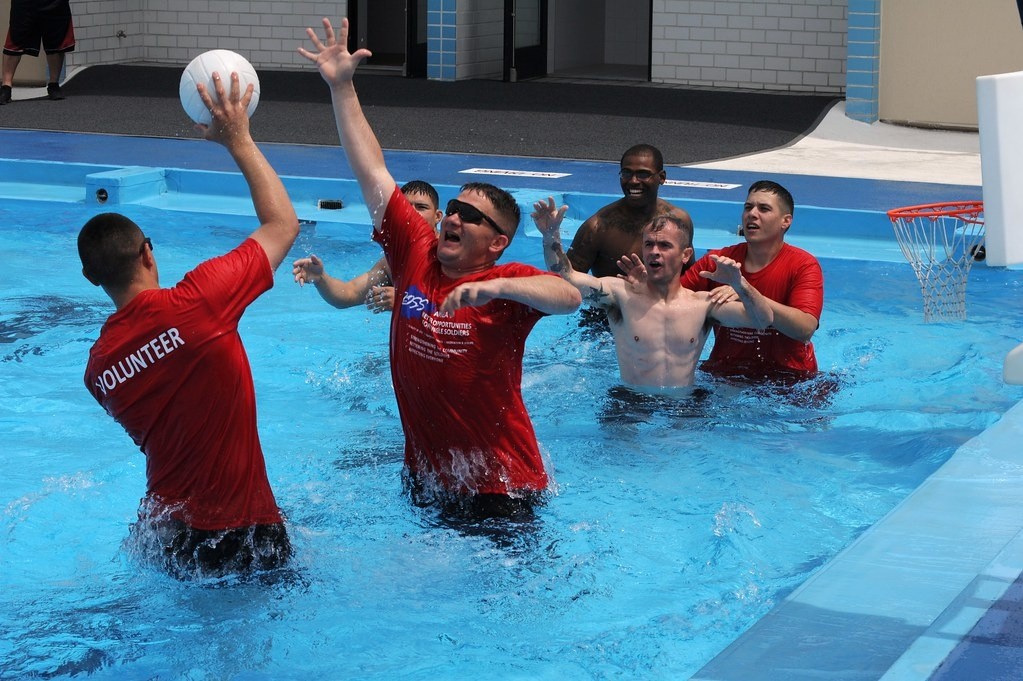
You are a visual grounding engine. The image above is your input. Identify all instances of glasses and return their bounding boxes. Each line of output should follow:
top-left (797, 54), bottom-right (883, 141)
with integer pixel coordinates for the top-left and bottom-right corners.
top-left (140, 237), bottom-right (153, 252)
top-left (618, 167), bottom-right (662, 181)
top-left (445, 199), bottom-right (506, 236)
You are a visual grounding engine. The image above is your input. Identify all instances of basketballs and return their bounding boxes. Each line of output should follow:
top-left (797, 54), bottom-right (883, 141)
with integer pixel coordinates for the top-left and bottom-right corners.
top-left (179, 48), bottom-right (261, 127)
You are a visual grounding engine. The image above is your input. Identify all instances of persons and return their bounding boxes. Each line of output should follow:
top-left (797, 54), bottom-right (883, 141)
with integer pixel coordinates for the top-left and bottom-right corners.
top-left (294, 14), bottom-right (581, 516)
top-left (566, 143), bottom-right (699, 278)
top-left (77, 71), bottom-right (304, 585)
top-left (0, 0), bottom-right (76, 104)
top-left (618, 179), bottom-right (824, 385)
top-left (531, 196), bottom-right (776, 398)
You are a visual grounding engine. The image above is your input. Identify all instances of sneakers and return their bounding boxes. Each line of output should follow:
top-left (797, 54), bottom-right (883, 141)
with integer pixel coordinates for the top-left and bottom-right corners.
top-left (0, 85), bottom-right (12, 104)
top-left (47, 82), bottom-right (65, 98)
